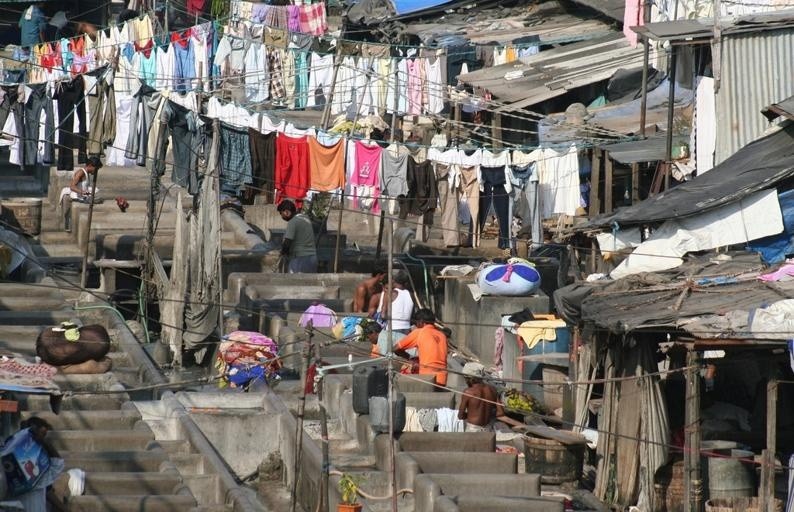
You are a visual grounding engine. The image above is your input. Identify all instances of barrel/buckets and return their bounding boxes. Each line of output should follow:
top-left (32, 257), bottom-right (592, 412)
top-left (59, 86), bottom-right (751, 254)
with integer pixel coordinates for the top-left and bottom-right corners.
top-left (708, 446), bottom-right (754, 498)
top-left (522, 324), bottom-right (571, 409)
top-left (1, 197), bottom-right (44, 235)
top-left (703, 497), bottom-right (785, 512)
top-left (353, 366), bottom-right (399, 414)
top-left (502, 326), bottom-right (523, 388)
top-left (665, 458), bottom-right (685, 512)
top-left (701, 439), bottom-right (752, 451)
top-left (524, 431), bottom-right (588, 491)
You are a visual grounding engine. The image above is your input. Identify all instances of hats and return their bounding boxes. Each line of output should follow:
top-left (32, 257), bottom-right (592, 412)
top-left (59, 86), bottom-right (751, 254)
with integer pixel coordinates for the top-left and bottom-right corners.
top-left (461, 361), bottom-right (486, 379)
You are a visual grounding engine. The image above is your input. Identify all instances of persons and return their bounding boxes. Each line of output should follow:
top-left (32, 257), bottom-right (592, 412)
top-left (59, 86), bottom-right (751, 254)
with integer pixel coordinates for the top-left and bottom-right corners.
top-left (351, 267), bottom-right (448, 394)
top-left (49, 9), bottom-right (76, 47)
top-left (457, 361), bottom-right (505, 433)
top-left (65, 155), bottom-right (104, 233)
top-left (73, 20), bottom-right (97, 41)
top-left (276, 199), bottom-right (320, 275)
top-left (4, 416), bottom-right (60, 492)
top-left (17, 1), bottom-right (46, 47)
